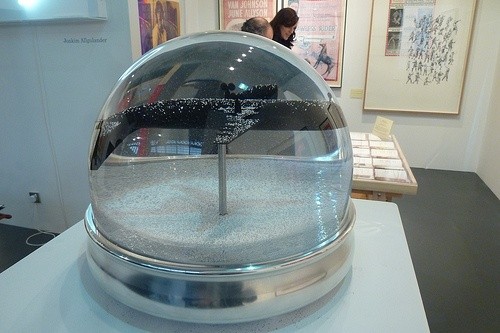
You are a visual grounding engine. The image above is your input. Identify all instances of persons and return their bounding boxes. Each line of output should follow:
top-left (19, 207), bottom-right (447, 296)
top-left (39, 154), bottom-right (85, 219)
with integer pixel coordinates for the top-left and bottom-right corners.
top-left (241, 17), bottom-right (273, 39)
top-left (269, 7), bottom-right (299, 50)
top-left (152, 1), bottom-right (167, 47)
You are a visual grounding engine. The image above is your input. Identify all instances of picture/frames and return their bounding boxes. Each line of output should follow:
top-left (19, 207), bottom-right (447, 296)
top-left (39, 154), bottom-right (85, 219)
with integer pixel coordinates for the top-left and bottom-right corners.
top-left (363, 0), bottom-right (477, 117)
top-left (282, 0), bottom-right (348, 89)
top-left (218, 0), bottom-right (279, 32)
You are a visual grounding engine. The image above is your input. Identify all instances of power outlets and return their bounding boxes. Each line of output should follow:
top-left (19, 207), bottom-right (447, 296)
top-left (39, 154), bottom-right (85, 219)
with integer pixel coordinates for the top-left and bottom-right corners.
top-left (28, 192), bottom-right (40, 203)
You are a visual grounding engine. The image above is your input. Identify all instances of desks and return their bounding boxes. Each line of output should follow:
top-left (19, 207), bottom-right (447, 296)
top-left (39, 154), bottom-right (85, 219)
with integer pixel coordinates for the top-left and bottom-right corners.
top-left (1, 198), bottom-right (427, 333)
